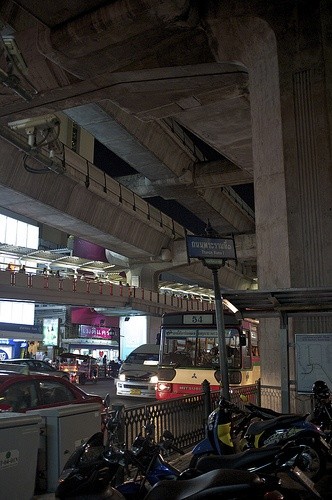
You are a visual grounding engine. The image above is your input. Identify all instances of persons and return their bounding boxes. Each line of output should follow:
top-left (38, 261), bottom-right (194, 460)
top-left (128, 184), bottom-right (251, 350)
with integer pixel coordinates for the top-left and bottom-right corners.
top-left (177, 340), bottom-right (193, 366)
top-left (103, 355), bottom-right (108, 366)
top-left (42, 352), bottom-right (47, 361)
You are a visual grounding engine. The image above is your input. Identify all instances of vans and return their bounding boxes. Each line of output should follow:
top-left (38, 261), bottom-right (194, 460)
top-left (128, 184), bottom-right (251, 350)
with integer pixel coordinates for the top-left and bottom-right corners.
top-left (114, 342), bottom-right (161, 402)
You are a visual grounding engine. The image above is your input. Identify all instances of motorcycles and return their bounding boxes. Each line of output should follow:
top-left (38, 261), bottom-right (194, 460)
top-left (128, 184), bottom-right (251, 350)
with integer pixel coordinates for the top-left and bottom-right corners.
top-left (58, 352), bottom-right (99, 385)
top-left (54, 389), bottom-right (332, 500)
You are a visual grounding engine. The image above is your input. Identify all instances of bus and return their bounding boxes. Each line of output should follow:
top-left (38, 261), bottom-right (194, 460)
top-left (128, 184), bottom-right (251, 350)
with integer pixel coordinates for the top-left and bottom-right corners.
top-left (154, 309), bottom-right (258, 409)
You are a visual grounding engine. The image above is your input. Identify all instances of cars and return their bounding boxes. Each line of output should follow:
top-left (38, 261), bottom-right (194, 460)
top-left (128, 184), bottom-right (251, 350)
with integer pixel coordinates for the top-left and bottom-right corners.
top-left (0, 359), bottom-right (70, 382)
top-left (0, 363), bottom-right (102, 414)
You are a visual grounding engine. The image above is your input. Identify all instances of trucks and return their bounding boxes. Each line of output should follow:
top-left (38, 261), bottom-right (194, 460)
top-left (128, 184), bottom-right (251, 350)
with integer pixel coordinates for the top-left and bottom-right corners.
top-left (117, 314), bottom-right (161, 362)
